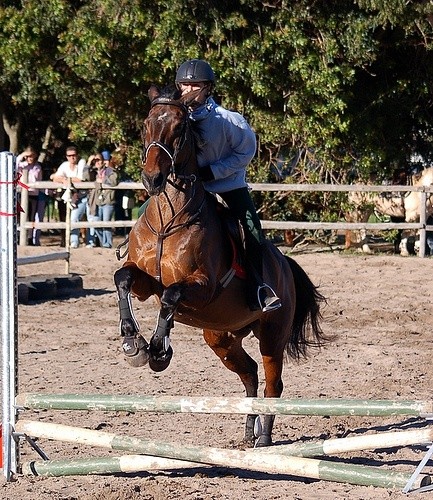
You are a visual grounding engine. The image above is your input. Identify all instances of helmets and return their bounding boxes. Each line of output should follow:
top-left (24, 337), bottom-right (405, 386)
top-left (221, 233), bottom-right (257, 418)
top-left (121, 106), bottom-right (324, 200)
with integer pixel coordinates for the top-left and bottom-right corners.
top-left (176, 60), bottom-right (217, 90)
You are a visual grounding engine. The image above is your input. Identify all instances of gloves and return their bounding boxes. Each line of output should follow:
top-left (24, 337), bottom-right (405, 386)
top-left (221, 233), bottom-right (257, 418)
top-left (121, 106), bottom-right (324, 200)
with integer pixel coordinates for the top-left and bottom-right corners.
top-left (197, 165), bottom-right (214, 181)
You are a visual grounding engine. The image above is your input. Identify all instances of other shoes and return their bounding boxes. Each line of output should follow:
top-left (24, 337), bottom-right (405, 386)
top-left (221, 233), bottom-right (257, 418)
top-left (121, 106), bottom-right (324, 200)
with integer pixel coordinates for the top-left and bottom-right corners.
top-left (256, 294), bottom-right (281, 311)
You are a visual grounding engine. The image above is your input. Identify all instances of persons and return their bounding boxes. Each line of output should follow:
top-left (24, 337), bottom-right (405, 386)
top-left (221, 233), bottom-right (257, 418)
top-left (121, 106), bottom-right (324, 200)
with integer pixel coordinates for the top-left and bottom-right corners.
top-left (136, 59), bottom-right (281, 312)
top-left (12, 147), bottom-right (117, 249)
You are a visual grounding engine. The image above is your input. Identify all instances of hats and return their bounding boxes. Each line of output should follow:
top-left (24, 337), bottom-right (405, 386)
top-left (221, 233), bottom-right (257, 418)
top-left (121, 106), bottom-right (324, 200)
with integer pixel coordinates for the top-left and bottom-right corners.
top-left (102, 151), bottom-right (110, 160)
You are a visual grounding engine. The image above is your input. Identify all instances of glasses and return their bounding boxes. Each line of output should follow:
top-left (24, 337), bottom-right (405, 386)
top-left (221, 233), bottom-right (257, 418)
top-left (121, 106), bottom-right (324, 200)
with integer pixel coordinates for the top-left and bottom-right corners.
top-left (66, 154), bottom-right (77, 156)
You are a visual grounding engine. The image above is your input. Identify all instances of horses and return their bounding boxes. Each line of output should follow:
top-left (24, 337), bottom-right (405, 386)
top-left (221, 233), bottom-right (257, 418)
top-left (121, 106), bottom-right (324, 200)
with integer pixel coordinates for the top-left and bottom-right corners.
top-left (345, 165), bottom-right (433, 257)
top-left (114, 82), bottom-right (340, 447)
